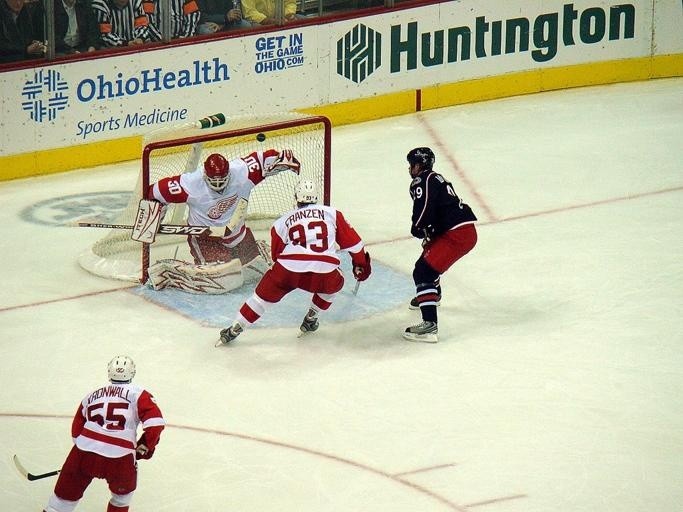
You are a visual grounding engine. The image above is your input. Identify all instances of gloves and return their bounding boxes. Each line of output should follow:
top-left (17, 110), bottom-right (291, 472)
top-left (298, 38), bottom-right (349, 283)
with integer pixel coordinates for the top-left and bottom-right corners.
top-left (352, 253), bottom-right (371, 281)
top-left (135, 435), bottom-right (153, 460)
top-left (411, 224), bottom-right (425, 238)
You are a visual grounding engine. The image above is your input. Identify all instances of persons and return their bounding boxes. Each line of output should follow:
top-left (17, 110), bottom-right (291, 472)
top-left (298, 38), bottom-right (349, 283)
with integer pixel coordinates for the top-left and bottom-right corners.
top-left (39, 358), bottom-right (163, 512)
top-left (219, 181), bottom-right (370, 344)
top-left (1, 0), bottom-right (310, 62)
top-left (404, 148), bottom-right (476, 333)
top-left (148, 150), bottom-right (300, 294)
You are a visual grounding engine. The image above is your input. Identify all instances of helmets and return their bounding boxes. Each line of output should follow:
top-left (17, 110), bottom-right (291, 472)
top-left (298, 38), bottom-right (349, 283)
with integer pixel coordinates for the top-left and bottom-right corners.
top-left (202, 154), bottom-right (231, 192)
top-left (108, 356), bottom-right (136, 381)
top-left (407, 147), bottom-right (435, 168)
top-left (294, 180), bottom-right (318, 204)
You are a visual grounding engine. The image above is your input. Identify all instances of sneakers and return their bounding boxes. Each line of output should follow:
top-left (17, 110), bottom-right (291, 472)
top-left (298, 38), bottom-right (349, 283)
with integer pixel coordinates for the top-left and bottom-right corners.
top-left (411, 294), bottom-right (441, 307)
top-left (406, 320), bottom-right (438, 334)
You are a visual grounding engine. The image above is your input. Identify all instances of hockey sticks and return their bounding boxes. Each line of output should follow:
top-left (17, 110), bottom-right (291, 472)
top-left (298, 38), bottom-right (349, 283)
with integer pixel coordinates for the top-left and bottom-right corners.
top-left (67, 197), bottom-right (248, 237)
top-left (13, 455), bottom-right (61, 481)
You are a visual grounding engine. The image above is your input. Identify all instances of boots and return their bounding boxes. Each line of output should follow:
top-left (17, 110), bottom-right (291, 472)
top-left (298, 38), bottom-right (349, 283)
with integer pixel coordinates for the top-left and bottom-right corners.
top-left (300, 308), bottom-right (319, 332)
top-left (220, 322), bottom-right (243, 344)
